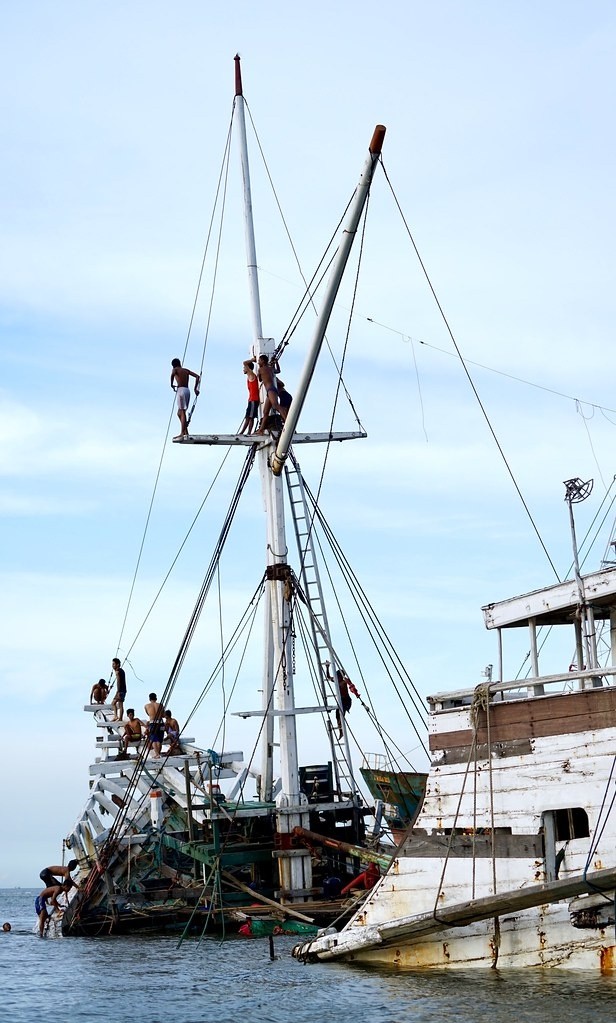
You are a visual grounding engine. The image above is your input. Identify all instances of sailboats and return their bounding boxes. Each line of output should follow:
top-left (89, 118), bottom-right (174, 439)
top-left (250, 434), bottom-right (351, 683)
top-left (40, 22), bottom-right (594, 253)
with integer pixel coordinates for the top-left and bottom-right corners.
top-left (60, 51), bottom-right (616, 993)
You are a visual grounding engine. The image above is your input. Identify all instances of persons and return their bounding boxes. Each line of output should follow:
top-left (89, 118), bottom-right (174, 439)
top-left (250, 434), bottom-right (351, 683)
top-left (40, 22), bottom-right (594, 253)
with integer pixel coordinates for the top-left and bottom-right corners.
top-left (89, 679), bottom-right (110, 706)
top-left (117, 708), bottom-right (149, 761)
top-left (143, 693), bottom-right (166, 759)
top-left (34, 879), bottom-right (73, 938)
top-left (256, 355), bottom-right (286, 434)
top-left (144, 721), bottom-right (154, 750)
top-left (164, 710), bottom-right (180, 757)
top-left (111, 658), bottom-right (127, 724)
top-left (170, 358), bottom-right (201, 439)
top-left (0, 921), bottom-right (11, 932)
top-left (276, 376), bottom-right (293, 423)
top-left (39, 859), bottom-right (81, 908)
top-left (239, 356), bottom-right (260, 437)
top-left (325, 658), bottom-right (355, 742)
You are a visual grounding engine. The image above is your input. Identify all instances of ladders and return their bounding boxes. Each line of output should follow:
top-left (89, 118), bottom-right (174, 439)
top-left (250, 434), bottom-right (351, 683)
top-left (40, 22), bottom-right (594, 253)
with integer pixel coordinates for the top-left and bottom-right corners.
top-left (283, 463), bottom-right (359, 827)
